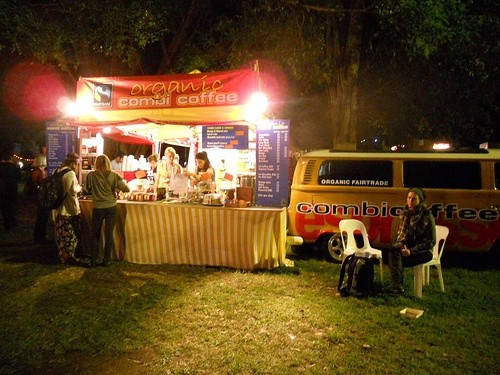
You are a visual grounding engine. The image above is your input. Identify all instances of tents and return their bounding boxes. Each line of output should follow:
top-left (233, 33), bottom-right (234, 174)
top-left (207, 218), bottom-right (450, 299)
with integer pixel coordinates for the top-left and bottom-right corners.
top-left (73, 66), bottom-right (282, 201)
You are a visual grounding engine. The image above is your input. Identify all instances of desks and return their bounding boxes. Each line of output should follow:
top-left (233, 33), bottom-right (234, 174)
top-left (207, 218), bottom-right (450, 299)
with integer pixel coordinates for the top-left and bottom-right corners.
top-left (79, 199), bottom-right (287, 269)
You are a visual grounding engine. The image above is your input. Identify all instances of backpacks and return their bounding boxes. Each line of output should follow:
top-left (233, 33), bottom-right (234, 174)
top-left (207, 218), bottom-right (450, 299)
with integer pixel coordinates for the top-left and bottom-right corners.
top-left (36, 166), bottom-right (74, 210)
top-left (337, 254), bottom-right (375, 299)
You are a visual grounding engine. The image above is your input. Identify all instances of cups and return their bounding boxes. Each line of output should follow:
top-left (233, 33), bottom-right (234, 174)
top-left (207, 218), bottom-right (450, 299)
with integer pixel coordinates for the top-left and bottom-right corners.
top-left (127, 194), bottom-right (156, 202)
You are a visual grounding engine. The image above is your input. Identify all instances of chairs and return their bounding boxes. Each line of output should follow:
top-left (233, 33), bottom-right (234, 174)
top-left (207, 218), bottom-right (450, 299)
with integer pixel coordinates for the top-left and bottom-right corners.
top-left (339, 219), bottom-right (383, 288)
top-left (408, 225), bottom-right (449, 298)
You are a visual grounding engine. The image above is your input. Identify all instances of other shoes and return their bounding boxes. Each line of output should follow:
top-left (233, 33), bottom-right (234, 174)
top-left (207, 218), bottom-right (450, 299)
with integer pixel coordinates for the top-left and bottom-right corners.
top-left (59, 256), bottom-right (111, 268)
top-left (384, 287), bottom-right (405, 296)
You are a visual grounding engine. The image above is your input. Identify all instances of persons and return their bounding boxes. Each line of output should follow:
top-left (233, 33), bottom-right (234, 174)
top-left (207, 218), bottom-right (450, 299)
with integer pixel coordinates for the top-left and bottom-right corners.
top-left (49, 153), bottom-right (83, 264)
top-left (85, 154), bottom-right (130, 267)
top-left (0, 156), bottom-right (18, 230)
top-left (111, 152), bottom-right (181, 173)
top-left (378, 186), bottom-right (437, 295)
top-left (155, 147), bottom-right (183, 191)
top-left (184, 151), bottom-right (217, 194)
top-left (31, 154), bottom-right (49, 244)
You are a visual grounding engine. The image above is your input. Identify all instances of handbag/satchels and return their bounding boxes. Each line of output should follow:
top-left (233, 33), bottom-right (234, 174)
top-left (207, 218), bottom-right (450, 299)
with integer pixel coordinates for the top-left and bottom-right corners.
top-left (23, 179), bottom-right (38, 195)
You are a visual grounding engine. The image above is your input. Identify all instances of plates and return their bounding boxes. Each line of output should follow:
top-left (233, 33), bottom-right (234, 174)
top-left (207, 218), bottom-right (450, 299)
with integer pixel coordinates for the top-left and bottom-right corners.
top-left (199, 202), bottom-right (224, 207)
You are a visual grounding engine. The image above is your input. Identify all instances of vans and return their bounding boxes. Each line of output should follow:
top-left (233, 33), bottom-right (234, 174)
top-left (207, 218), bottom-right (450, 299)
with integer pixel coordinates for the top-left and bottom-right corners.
top-left (287, 144), bottom-right (500, 264)
